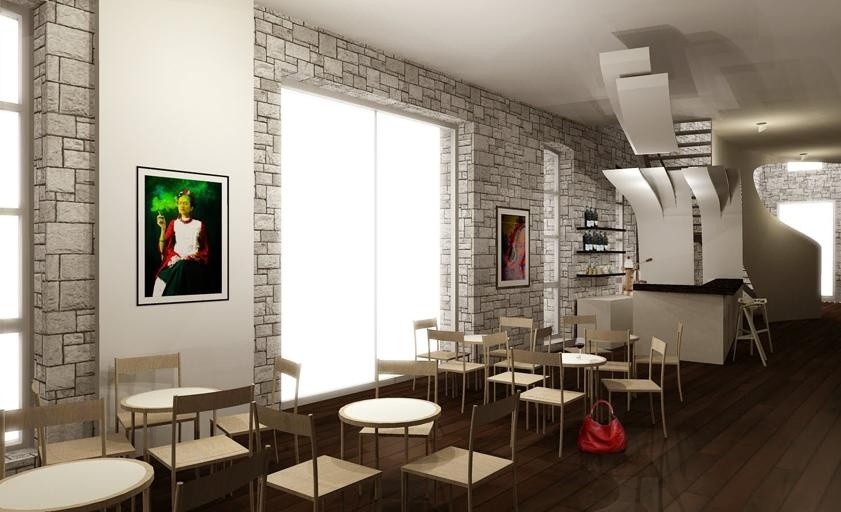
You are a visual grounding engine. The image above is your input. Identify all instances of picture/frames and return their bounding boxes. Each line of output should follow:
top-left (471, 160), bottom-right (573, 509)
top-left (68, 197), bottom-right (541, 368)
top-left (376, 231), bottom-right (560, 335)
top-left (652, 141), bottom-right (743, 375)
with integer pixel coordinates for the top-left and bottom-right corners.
top-left (495, 205), bottom-right (530, 290)
top-left (135, 166), bottom-right (230, 308)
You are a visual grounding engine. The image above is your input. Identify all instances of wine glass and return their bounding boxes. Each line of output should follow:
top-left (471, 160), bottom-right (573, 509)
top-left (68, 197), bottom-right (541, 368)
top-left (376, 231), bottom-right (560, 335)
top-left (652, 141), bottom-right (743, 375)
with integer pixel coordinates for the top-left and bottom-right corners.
top-left (587, 257), bottom-right (592, 275)
top-left (592, 257), bottom-right (598, 275)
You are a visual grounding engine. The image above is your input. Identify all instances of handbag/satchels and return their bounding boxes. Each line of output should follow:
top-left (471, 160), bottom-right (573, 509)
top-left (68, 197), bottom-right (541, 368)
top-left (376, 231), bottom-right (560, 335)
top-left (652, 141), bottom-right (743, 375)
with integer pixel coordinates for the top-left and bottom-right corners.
top-left (577, 399), bottom-right (627, 454)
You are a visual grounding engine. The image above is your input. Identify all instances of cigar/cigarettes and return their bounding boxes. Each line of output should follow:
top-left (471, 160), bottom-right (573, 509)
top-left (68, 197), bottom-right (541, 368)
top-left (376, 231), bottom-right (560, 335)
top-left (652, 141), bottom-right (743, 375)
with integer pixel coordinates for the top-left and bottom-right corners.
top-left (158, 211), bottom-right (161, 215)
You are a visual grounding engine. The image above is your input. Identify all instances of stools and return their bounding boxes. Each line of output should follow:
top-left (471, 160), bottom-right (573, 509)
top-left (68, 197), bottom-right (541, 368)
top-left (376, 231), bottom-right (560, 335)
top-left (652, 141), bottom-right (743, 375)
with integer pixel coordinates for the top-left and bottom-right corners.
top-left (737, 297), bottom-right (773, 354)
top-left (732, 303), bottom-right (764, 367)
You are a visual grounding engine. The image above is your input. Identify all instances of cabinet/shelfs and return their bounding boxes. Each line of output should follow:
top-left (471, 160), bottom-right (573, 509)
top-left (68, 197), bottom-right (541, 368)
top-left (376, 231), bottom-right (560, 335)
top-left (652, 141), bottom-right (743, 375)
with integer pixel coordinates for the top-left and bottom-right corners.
top-left (575, 226), bottom-right (627, 278)
top-left (575, 294), bottom-right (632, 343)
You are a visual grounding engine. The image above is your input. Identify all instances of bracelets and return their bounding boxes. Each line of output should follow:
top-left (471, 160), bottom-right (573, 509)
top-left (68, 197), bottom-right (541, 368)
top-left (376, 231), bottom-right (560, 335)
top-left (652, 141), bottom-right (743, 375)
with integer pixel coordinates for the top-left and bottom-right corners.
top-left (159, 239), bottom-right (165, 242)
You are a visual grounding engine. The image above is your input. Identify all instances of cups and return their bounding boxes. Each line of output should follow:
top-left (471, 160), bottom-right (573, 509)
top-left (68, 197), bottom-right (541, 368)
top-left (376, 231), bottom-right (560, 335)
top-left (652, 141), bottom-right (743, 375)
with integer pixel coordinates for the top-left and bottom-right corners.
top-left (609, 288), bottom-right (616, 295)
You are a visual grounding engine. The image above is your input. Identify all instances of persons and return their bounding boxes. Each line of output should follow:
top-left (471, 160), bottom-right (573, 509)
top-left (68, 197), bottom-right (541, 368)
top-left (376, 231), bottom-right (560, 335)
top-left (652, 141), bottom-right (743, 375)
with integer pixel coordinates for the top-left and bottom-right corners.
top-left (504, 216), bottom-right (525, 280)
top-left (152, 190), bottom-right (209, 297)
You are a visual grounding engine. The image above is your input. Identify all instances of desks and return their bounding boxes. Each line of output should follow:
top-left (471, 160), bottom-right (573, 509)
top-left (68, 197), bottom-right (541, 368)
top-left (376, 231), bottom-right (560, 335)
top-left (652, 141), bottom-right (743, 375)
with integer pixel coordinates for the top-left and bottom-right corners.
top-left (450, 334), bottom-right (509, 398)
top-left (543, 353), bottom-right (607, 437)
top-left (0, 458), bottom-right (155, 512)
top-left (575, 335), bottom-right (640, 400)
top-left (338, 397), bottom-right (442, 512)
top-left (120, 386), bottom-right (223, 512)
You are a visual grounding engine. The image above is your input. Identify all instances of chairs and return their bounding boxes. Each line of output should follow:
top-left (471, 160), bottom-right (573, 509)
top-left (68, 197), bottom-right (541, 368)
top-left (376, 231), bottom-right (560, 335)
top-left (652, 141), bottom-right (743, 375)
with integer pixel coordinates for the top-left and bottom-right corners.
top-left (426, 328), bottom-right (486, 414)
top-left (412, 318), bottom-right (470, 397)
top-left (114, 352), bottom-right (201, 483)
top-left (480, 331), bottom-right (551, 432)
top-left (633, 324), bottom-right (684, 404)
top-left (582, 328), bottom-right (631, 414)
top-left (357, 358), bottom-right (438, 503)
top-left (479, 316), bottom-right (534, 398)
top-left (400, 389), bottom-right (522, 512)
top-left (252, 401), bottom-right (383, 512)
top-left (31, 382), bottom-right (136, 512)
top-left (145, 383), bottom-right (255, 512)
top-left (599, 336), bottom-right (668, 440)
top-left (508, 347), bottom-right (586, 459)
top-left (209, 356), bottom-right (300, 500)
top-left (493, 325), bottom-right (553, 404)
top-left (561, 313), bottom-right (615, 399)
top-left (0, 397), bottom-right (106, 481)
